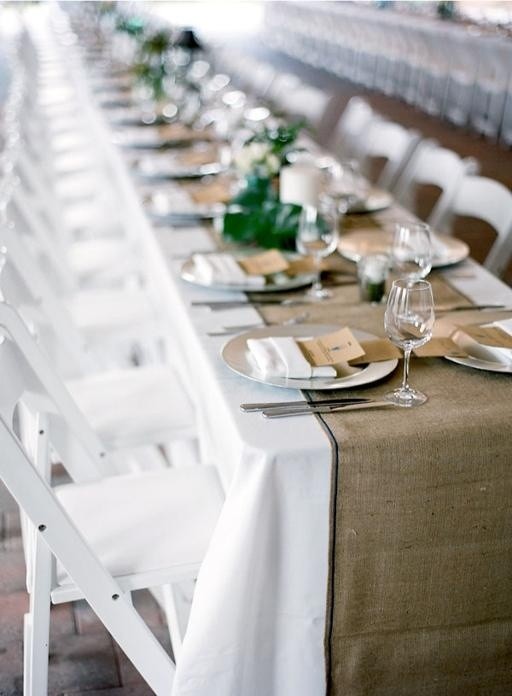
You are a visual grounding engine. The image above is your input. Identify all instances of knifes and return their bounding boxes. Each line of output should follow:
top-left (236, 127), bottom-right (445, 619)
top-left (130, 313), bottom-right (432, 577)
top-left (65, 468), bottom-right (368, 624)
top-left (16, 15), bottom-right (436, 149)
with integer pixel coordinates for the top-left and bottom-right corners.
top-left (239, 394), bottom-right (396, 422)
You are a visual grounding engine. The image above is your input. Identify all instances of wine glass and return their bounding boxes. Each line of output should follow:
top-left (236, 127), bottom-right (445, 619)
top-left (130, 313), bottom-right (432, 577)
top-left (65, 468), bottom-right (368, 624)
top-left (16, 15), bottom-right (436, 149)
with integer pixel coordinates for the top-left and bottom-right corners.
top-left (383, 279), bottom-right (436, 409)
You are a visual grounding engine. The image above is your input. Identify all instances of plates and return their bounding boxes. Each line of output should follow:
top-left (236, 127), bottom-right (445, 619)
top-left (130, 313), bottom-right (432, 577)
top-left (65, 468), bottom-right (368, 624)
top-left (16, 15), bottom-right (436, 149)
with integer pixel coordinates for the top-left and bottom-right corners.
top-left (56, 0), bottom-right (511, 392)
